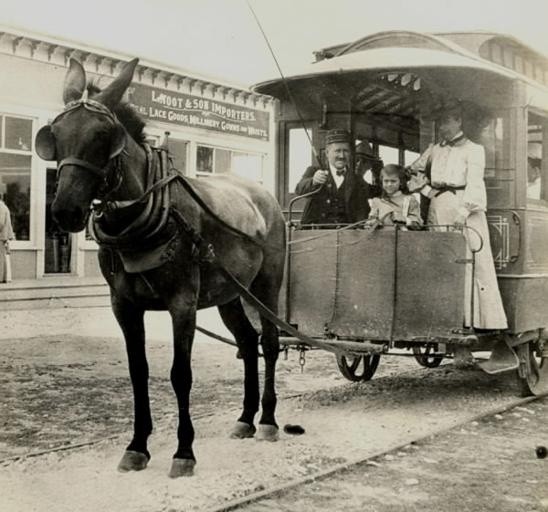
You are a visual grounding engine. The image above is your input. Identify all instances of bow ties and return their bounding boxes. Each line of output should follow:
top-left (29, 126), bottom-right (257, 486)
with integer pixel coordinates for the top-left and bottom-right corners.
top-left (336, 170), bottom-right (345, 176)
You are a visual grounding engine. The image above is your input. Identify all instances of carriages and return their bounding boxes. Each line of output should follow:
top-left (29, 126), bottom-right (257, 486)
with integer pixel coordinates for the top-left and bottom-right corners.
top-left (35, 30), bottom-right (548, 480)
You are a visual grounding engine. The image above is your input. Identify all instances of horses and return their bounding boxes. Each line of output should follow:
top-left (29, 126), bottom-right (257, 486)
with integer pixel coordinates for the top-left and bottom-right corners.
top-left (33, 55), bottom-right (288, 479)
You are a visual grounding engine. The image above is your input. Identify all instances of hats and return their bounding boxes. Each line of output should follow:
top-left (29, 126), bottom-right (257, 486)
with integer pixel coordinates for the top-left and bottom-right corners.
top-left (356, 140), bottom-right (379, 160)
top-left (325, 128), bottom-right (350, 145)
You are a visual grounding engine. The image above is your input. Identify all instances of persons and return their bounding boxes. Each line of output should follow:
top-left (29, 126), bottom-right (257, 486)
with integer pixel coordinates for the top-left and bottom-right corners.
top-left (367, 164), bottom-right (424, 231)
top-left (526, 157), bottom-right (542, 199)
top-left (411, 106), bottom-right (508, 330)
top-left (0, 192), bottom-right (14, 282)
top-left (295, 128), bottom-right (370, 231)
top-left (354, 140), bottom-right (383, 198)
